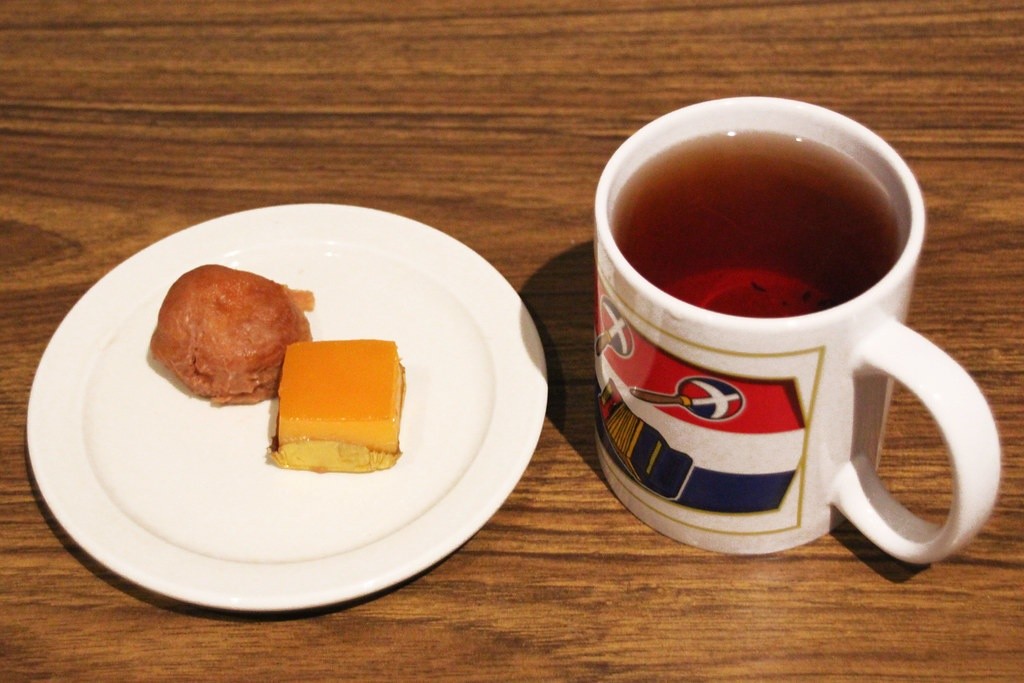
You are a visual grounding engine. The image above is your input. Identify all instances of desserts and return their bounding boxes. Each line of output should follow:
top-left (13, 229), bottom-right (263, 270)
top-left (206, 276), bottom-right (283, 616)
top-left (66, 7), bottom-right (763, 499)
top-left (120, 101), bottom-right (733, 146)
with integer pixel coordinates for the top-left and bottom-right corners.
top-left (150, 263), bottom-right (312, 407)
top-left (269, 339), bottom-right (406, 469)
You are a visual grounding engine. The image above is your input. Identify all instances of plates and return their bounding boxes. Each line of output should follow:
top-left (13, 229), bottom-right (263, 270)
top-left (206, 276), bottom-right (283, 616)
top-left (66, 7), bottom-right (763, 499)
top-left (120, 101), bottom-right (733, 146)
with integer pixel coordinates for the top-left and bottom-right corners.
top-left (26, 203), bottom-right (548, 611)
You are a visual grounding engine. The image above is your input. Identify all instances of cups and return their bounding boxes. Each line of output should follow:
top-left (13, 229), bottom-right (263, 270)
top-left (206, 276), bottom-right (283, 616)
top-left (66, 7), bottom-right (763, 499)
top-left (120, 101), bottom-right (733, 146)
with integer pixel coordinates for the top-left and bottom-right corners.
top-left (593, 96), bottom-right (1002, 564)
